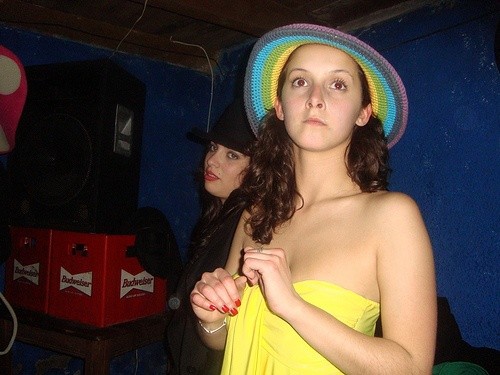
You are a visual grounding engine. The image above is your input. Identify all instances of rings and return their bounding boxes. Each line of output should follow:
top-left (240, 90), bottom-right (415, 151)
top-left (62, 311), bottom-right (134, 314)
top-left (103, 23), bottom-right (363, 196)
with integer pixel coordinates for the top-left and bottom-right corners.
top-left (258, 248), bottom-right (262, 253)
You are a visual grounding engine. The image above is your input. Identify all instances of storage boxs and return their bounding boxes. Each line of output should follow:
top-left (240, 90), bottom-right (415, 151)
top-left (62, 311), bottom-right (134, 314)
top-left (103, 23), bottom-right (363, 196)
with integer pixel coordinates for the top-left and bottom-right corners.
top-left (4, 222), bottom-right (53, 318)
top-left (48, 226), bottom-right (172, 332)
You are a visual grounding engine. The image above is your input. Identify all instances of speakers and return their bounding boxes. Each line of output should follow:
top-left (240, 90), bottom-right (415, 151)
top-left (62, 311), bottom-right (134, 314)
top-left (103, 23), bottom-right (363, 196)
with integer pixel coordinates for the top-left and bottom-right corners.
top-left (8, 59), bottom-right (147, 236)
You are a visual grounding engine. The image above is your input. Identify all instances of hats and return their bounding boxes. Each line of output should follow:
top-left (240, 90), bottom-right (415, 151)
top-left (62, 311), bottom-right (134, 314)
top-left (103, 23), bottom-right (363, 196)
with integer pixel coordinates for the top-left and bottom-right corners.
top-left (185, 108), bottom-right (257, 157)
top-left (243, 24), bottom-right (408, 148)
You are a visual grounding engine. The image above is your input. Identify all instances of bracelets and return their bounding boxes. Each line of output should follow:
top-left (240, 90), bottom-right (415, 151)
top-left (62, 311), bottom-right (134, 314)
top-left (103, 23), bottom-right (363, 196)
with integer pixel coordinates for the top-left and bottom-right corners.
top-left (198, 318), bottom-right (227, 336)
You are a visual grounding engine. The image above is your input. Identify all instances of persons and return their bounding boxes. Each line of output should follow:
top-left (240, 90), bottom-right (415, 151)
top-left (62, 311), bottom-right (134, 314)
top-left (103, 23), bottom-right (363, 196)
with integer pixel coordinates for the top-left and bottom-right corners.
top-left (166, 100), bottom-right (258, 375)
top-left (190, 23), bottom-right (438, 375)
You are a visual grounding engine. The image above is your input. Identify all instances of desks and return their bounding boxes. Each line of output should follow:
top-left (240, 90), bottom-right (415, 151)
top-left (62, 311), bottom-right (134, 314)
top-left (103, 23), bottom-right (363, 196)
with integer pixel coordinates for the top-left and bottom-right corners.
top-left (1, 317), bottom-right (178, 375)
